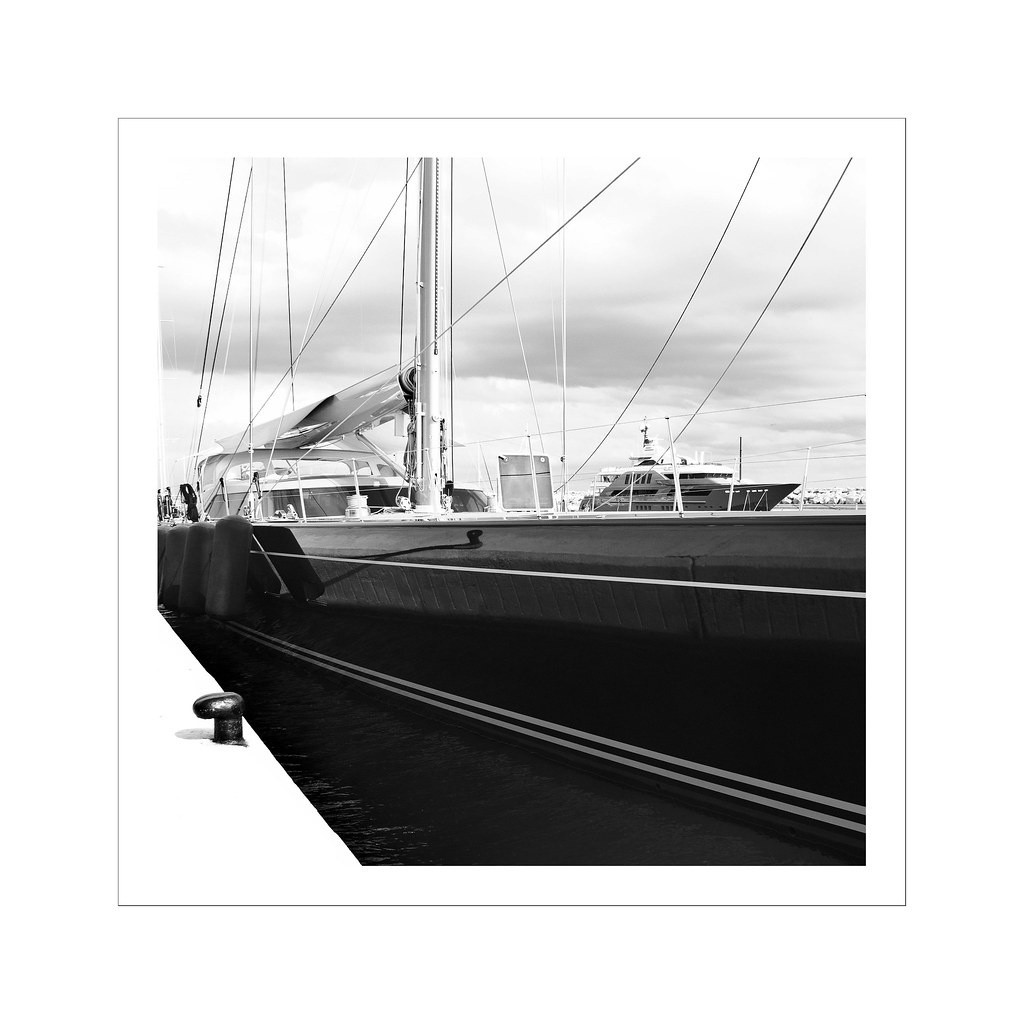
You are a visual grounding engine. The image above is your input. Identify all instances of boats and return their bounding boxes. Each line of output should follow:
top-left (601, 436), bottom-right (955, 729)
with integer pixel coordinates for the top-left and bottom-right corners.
top-left (153, 153), bottom-right (866, 663)
